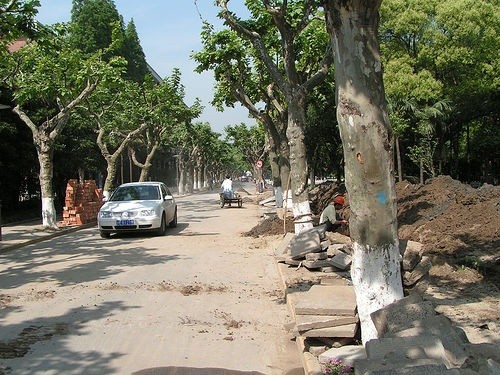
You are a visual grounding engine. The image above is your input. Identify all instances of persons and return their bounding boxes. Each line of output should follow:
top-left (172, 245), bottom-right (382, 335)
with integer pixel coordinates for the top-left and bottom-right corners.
top-left (207, 176), bottom-right (213, 191)
top-left (221, 175), bottom-right (233, 206)
top-left (319, 196), bottom-right (347, 232)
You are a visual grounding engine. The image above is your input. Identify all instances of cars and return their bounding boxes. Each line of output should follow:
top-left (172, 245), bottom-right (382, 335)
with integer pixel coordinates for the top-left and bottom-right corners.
top-left (97, 182), bottom-right (178, 239)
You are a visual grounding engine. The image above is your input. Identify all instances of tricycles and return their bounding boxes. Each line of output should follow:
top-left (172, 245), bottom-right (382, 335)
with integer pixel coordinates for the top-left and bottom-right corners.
top-left (218, 186), bottom-right (243, 208)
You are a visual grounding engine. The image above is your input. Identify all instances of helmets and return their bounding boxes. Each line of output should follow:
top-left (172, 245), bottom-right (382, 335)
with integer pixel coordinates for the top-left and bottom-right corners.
top-left (334, 196), bottom-right (344, 206)
